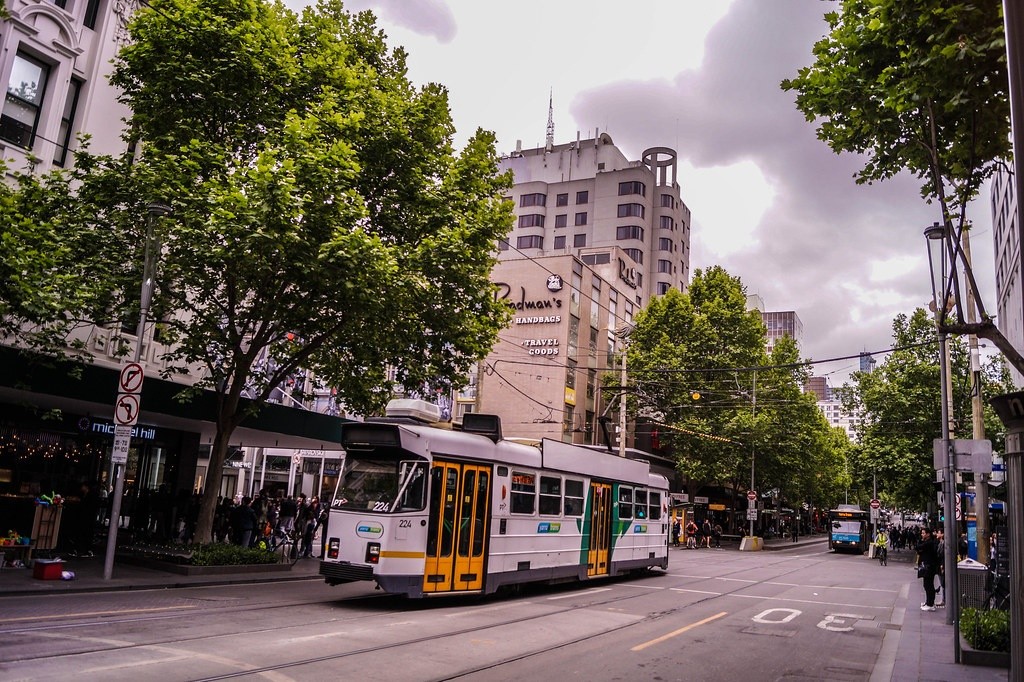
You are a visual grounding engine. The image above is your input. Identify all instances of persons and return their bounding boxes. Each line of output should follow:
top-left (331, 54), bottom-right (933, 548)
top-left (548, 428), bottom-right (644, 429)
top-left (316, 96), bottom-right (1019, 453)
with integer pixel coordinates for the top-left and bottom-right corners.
top-left (671, 517), bottom-right (824, 549)
top-left (98, 477), bottom-right (327, 559)
top-left (871, 520), bottom-right (996, 611)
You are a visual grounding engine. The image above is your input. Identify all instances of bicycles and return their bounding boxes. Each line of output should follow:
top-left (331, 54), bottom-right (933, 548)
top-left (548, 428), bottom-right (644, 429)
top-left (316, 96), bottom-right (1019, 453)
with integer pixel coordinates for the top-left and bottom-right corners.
top-left (876, 544), bottom-right (888, 565)
top-left (255, 528), bottom-right (300, 567)
top-left (689, 535), bottom-right (704, 550)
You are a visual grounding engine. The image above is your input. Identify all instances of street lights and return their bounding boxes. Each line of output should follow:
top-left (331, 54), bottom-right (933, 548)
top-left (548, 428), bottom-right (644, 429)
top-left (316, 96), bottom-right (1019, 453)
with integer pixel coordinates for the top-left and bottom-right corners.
top-left (102, 203), bottom-right (173, 580)
top-left (922, 221), bottom-right (955, 626)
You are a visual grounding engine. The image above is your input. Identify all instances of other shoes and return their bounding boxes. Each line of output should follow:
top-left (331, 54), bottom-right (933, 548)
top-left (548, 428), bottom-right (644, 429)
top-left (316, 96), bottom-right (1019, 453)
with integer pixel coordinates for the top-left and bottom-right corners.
top-left (936, 600), bottom-right (945, 607)
top-left (920, 603), bottom-right (936, 612)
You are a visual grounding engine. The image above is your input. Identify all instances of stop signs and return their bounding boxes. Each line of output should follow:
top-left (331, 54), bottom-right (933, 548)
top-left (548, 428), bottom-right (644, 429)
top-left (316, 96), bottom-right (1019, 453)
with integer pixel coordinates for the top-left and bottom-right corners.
top-left (870, 499), bottom-right (880, 509)
top-left (747, 491), bottom-right (756, 500)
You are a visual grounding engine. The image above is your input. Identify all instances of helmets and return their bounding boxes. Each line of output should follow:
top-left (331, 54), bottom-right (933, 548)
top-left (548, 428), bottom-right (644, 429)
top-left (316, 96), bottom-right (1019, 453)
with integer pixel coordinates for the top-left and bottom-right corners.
top-left (880, 528), bottom-right (885, 532)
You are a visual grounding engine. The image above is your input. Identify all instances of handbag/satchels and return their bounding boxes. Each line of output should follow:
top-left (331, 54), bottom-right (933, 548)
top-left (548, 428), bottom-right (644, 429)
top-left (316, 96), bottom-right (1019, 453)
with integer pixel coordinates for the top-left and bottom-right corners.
top-left (918, 564), bottom-right (939, 578)
top-left (678, 533), bottom-right (681, 537)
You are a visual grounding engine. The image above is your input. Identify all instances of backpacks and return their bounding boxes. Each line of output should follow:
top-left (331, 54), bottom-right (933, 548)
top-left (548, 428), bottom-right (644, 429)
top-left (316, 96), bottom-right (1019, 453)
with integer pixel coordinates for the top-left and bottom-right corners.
top-left (687, 523), bottom-right (694, 532)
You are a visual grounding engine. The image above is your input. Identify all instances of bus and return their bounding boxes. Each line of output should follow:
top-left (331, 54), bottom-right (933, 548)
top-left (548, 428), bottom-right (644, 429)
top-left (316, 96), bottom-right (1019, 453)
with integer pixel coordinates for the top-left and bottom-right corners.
top-left (318, 385), bottom-right (671, 600)
top-left (828, 504), bottom-right (871, 554)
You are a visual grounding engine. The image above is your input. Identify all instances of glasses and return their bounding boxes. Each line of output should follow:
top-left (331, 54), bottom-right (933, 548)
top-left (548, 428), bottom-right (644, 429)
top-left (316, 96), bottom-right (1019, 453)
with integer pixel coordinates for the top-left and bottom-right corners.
top-left (263, 495), bottom-right (270, 498)
